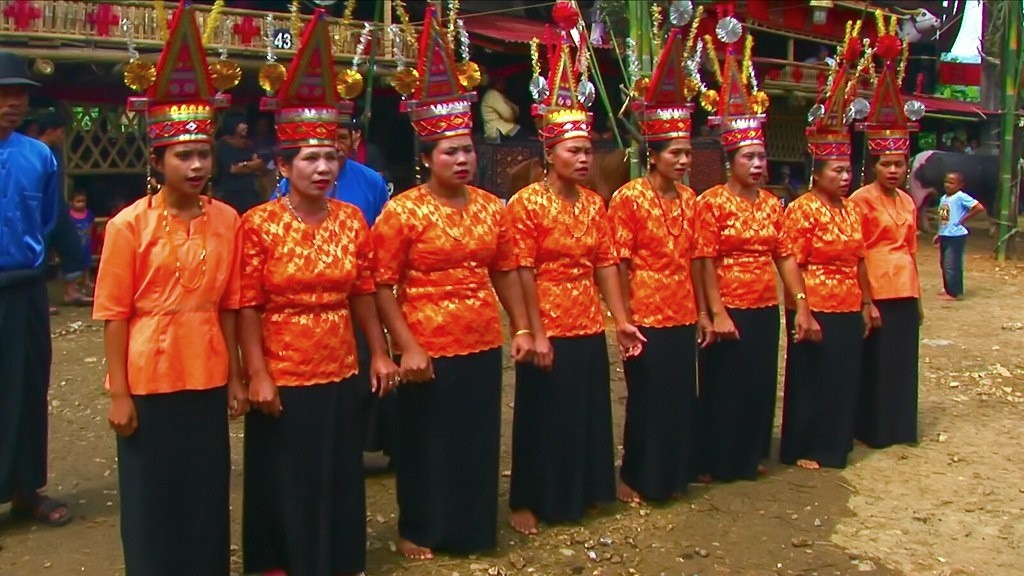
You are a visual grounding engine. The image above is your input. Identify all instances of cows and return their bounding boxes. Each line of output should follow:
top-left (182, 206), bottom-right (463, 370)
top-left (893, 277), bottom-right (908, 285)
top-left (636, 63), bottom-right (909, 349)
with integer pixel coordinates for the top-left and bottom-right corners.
top-left (893, 5), bottom-right (942, 44)
top-left (906, 150), bottom-right (1018, 239)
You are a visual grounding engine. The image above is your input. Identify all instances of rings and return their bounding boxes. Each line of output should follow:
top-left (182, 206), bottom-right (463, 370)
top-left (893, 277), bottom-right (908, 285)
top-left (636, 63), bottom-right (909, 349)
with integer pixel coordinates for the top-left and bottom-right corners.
top-left (389, 376), bottom-right (401, 383)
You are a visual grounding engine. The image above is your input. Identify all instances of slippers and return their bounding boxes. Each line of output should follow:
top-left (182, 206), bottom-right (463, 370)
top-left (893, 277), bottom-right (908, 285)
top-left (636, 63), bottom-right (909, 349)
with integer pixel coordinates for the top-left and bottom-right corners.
top-left (10, 493), bottom-right (74, 526)
top-left (63, 292), bottom-right (96, 307)
top-left (48, 300), bottom-right (58, 317)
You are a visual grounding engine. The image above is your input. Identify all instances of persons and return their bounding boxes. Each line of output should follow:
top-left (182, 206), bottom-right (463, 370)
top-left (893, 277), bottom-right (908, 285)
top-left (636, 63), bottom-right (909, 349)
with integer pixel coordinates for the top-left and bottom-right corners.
top-left (241, 0), bottom-right (402, 576)
top-left (779, 0), bottom-right (871, 473)
top-left (608, 0), bottom-right (714, 503)
top-left (931, 171), bottom-right (984, 301)
top-left (695, 0), bottom-right (811, 480)
top-left (19, 110), bottom-right (385, 308)
top-left (370, 0), bottom-right (534, 559)
top-left (938, 134), bottom-right (979, 153)
top-left (507, 1), bottom-right (647, 535)
top-left (848, 0), bottom-right (925, 452)
top-left (90, 0), bottom-right (243, 576)
top-left (271, 99), bottom-right (391, 483)
top-left (1, 52), bottom-right (73, 528)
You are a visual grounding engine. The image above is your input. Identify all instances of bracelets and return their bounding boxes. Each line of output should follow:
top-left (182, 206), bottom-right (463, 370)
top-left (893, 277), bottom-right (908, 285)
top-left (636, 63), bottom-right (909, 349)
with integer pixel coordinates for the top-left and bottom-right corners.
top-left (698, 311), bottom-right (708, 318)
top-left (862, 302), bottom-right (871, 305)
top-left (792, 291), bottom-right (807, 301)
top-left (511, 330), bottom-right (535, 338)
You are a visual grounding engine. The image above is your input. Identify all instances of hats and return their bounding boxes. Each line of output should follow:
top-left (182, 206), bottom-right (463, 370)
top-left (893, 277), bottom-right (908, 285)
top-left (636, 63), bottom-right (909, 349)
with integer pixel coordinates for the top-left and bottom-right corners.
top-left (274, 0), bottom-right (342, 150)
top-left (636, 0), bottom-right (694, 141)
top-left (864, 6), bottom-right (913, 156)
top-left (804, 19), bottom-right (870, 159)
top-left (409, 0), bottom-right (475, 141)
top-left (0, 53), bottom-right (42, 86)
top-left (145, 0), bottom-right (219, 146)
top-left (531, 0), bottom-right (594, 148)
top-left (712, 0), bottom-right (769, 150)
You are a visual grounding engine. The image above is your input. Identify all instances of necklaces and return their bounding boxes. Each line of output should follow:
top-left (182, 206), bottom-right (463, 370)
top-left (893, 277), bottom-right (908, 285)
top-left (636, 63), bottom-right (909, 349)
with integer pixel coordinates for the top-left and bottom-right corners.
top-left (545, 183), bottom-right (589, 238)
top-left (647, 177), bottom-right (685, 237)
top-left (726, 183), bottom-right (764, 230)
top-left (877, 190), bottom-right (908, 226)
top-left (810, 190), bottom-right (853, 237)
top-left (160, 190), bottom-right (208, 292)
top-left (427, 186), bottom-right (467, 241)
top-left (285, 194), bottom-right (336, 265)
